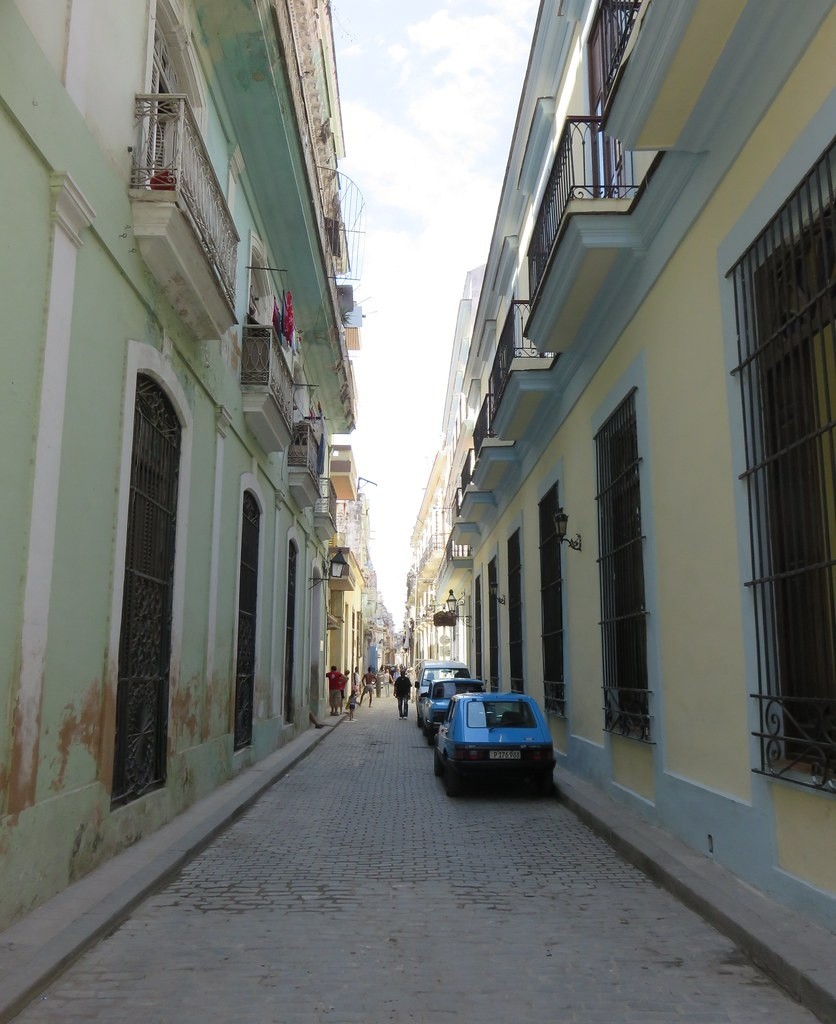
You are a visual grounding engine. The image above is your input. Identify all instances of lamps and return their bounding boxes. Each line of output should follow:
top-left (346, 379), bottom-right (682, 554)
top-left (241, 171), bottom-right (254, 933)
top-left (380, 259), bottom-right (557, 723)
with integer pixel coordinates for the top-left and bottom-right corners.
top-left (489, 579), bottom-right (505, 604)
top-left (446, 589), bottom-right (472, 627)
top-left (553, 507), bottom-right (582, 551)
top-left (306, 548), bottom-right (347, 590)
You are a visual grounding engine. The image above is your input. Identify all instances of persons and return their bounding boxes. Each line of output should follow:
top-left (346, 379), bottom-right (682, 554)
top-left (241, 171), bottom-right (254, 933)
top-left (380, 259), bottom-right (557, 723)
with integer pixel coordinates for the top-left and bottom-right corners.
top-left (327, 663), bottom-right (412, 721)
top-left (308, 710), bottom-right (325, 729)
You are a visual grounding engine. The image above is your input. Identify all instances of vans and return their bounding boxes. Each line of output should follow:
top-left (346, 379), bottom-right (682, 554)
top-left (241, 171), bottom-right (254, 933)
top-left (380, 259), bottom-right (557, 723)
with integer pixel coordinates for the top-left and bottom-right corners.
top-left (414, 661), bottom-right (471, 728)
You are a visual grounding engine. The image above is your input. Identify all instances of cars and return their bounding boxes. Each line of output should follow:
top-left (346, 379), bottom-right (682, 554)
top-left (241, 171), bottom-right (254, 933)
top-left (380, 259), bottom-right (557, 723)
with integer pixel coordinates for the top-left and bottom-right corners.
top-left (432, 691), bottom-right (557, 798)
top-left (421, 677), bottom-right (496, 745)
top-left (380, 663), bottom-right (399, 683)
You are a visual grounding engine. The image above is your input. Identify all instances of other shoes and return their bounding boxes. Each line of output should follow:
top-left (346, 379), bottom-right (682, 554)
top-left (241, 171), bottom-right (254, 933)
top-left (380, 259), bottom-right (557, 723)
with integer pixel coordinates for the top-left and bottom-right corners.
top-left (403, 717), bottom-right (407, 719)
top-left (399, 717), bottom-right (402, 719)
top-left (331, 712), bottom-right (339, 715)
top-left (316, 724), bottom-right (323, 728)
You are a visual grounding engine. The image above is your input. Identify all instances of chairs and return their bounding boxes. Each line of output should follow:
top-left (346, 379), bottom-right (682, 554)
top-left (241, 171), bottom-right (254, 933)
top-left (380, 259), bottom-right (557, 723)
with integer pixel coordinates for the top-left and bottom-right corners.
top-left (497, 711), bottom-right (525, 727)
